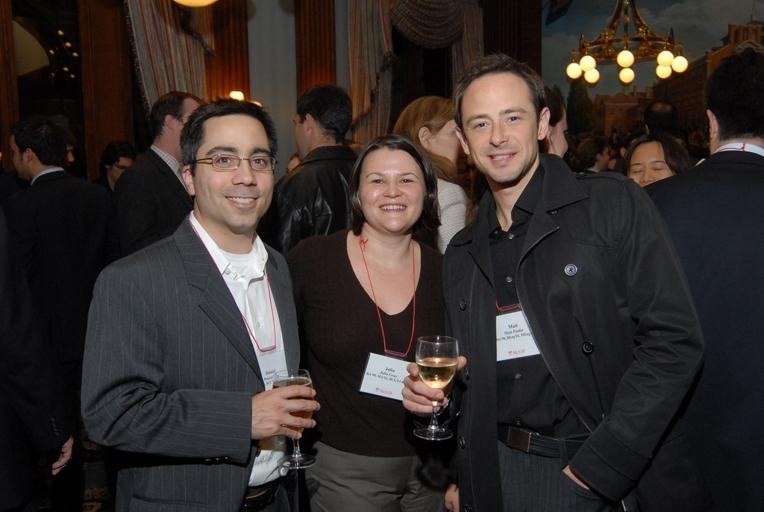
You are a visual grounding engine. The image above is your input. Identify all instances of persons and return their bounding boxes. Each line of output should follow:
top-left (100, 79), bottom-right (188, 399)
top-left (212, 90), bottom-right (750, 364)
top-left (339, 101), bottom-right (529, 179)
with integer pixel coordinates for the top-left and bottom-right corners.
top-left (400, 54), bottom-right (704, 510)
top-left (630, 46), bottom-right (764, 512)
top-left (2, 87), bottom-right (764, 509)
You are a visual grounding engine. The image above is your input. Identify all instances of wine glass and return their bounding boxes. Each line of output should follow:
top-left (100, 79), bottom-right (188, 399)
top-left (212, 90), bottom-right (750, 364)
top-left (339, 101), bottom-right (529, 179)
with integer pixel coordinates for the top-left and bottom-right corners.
top-left (413, 335), bottom-right (460, 441)
top-left (271, 369), bottom-right (319, 472)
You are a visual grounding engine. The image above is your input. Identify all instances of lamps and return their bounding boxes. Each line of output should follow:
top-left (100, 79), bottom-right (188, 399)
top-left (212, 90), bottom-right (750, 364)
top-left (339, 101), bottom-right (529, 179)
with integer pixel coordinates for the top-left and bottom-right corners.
top-left (565, 1), bottom-right (689, 85)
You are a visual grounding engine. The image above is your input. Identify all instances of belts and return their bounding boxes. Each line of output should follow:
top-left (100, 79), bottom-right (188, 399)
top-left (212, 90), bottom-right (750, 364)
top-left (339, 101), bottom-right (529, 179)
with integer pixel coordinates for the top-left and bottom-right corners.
top-left (241, 483), bottom-right (281, 511)
top-left (497, 422), bottom-right (592, 459)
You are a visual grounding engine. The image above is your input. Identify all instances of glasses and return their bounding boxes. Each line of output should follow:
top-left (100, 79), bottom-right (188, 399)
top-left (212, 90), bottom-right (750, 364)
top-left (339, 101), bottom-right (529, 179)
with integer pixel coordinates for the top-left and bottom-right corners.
top-left (187, 153), bottom-right (277, 172)
